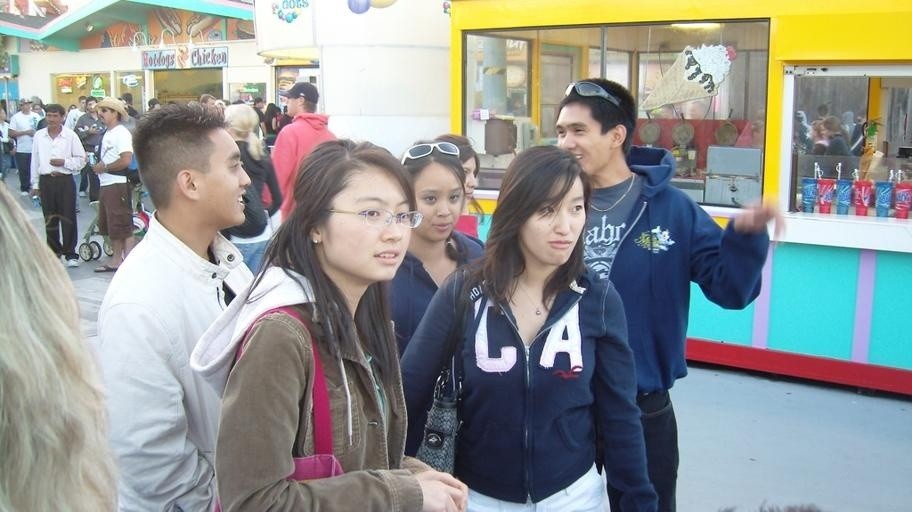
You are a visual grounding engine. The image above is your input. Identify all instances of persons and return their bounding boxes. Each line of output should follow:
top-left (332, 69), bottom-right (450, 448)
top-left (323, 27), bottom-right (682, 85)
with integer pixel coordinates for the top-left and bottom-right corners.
top-left (796, 105), bottom-right (868, 174)
top-left (94, 104), bottom-right (260, 511)
top-left (0, 183), bottom-right (120, 512)
top-left (401, 146), bottom-right (664, 512)
top-left (387, 144), bottom-right (487, 349)
top-left (147, 83), bottom-right (337, 278)
top-left (436, 135), bottom-right (479, 202)
top-left (190, 139), bottom-right (471, 511)
top-left (0, 94), bottom-right (141, 271)
top-left (556, 79), bottom-right (788, 511)
top-left (662, 103), bottom-right (675, 119)
top-left (688, 100), bottom-right (707, 121)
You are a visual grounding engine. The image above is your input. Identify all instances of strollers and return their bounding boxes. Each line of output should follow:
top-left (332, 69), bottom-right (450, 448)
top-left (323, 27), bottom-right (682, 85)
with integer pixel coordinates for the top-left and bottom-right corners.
top-left (79, 178), bottom-right (153, 262)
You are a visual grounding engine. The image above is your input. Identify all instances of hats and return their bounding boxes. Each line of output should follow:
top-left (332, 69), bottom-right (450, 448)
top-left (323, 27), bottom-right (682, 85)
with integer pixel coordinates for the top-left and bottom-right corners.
top-left (280, 82), bottom-right (318, 103)
top-left (95, 97), bottom-right (128, 121)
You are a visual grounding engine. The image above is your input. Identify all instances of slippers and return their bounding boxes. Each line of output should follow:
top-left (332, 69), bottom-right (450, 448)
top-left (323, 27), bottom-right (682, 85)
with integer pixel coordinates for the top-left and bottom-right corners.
top-left (95, 265), bottom-right (117, 272)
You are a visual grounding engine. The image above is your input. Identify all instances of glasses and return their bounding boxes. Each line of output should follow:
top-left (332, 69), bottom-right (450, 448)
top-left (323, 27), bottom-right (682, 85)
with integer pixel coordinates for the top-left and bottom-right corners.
top-left (401, 142), bottom-right (460, 164)
top-left (329, 209), bottom-right (424, 228)
top-left (565, 82), bottom-right (620, 107)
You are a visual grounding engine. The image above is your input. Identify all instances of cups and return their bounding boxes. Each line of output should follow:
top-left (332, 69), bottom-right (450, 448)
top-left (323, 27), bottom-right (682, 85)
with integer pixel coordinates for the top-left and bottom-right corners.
top-left (873, 181), bottom-right (891, 217)
top-left (856, 180), bottom-right (872, 216)
top-left (836, 180), bottom-right (852, 214)
top-left (800, 178), bottom-right (817, 214)
top-left (32, 196), bottom-right (41, 208)
top-left (893, 182), bottom-right (911, 218)
top-left (817, 179), bottom-right (835, 214)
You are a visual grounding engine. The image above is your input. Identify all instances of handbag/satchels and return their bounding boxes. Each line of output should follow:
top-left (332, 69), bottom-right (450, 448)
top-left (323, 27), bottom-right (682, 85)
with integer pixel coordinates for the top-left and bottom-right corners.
top-left (415, 370), bottom-right (463, 474)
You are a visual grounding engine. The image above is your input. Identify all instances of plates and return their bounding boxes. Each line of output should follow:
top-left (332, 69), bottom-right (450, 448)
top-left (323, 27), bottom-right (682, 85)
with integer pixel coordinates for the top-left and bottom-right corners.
top-left (639, 120), bottom-right (660, 144)
top-left (715, 122), bottom-right (737, 146)
top-left (672, 122), bottom-right (695, 144)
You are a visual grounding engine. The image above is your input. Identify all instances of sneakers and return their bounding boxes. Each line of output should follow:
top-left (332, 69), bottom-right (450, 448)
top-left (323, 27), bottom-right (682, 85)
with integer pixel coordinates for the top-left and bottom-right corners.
top-left (68, 259), bottom-right (80, 267)
